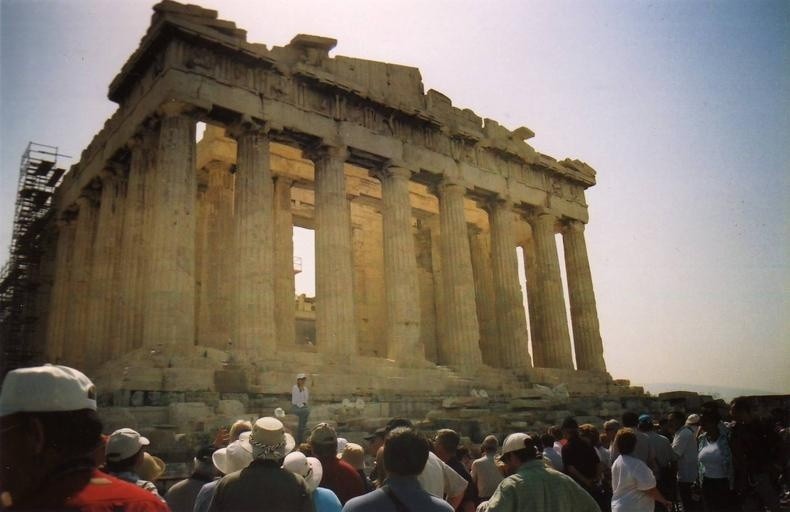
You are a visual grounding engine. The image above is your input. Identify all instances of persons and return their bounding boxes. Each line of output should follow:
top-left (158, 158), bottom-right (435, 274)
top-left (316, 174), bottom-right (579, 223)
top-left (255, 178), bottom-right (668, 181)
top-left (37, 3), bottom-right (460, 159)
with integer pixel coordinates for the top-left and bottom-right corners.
top-left (0, 364), bottom-right (169, 511)
top-left (769, 407), bottom-right (786, 497)
top-left (604, 419), bottom-right (619, 442)
top-left (635, 414), bottom-right (679, 510)
top-left (430, 428), bottom-right (477, 510)
top-left (478, 433), bottom-right (603, 512)
top-left (597, 433), bottom-right (610, 453)
top-left (195, 445), bottom-right (254, 510)
top-left (301, 422), bottom-right (364, 504)
top-left (292, 373), bottom-right (309, 440)
top-left (728, 395), bottom-right (776, 511)
top-left (211, 419), bottom-right (253, 448)
top-left (373, 443), bottom-right (388, 474)
top-left (161, 443), bottom-right (221, 511)
top-left (610, 431), bottom-right (673, 510)
top-left (344, 427), bottom-right (453, 512)
top-left (610, 412), bottom-right (658, 476)
top-left (333, 438), bottom-right (350, 462)
top-left (98, 428), bottom-right (163, 499)
top-left (576, 424), bottom-right (610, 474)
top-left (666, 412), bottom-right (700, 509)
top-left (699, 401), bottom-right (728, 450)
top-left (469, 436), bottom-right (509, 502)
top-left (386, 418), bottom-right (467, 512)
top-left (690, 418), bottom-right (734, 511)
top-left (281, 452), bottom-right (342, 512)
top-left (338, 443), bottom-right (368, 496)
top-left (210, 414), bottom-right (314, 511)
top-left (363, 429), bottom-right (391, 484)
top-left (659, 417), bottom-right (674, 442)
top-left (131, 450), bottom-right (166, 486)
top-left (559, 415), bottom-right (602, 496)
top-left (538, 432), bottom-right (562, 472)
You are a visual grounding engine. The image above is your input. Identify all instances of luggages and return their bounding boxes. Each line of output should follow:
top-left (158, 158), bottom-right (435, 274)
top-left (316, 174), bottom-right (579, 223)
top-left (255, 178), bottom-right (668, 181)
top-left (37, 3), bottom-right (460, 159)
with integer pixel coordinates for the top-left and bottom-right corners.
top-left (496, 432), bottom-right (539, 462)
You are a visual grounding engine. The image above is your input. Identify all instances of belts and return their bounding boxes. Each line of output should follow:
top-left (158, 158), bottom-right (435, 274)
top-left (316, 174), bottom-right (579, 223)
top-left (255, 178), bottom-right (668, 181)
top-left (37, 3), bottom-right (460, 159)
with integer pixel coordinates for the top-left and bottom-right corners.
top-left (477, 497), bottom-right (489, 500)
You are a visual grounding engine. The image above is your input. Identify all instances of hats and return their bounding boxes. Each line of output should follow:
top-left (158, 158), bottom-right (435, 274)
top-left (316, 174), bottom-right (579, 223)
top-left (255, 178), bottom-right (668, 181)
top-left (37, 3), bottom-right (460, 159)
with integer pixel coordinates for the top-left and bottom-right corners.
top-left (137, 451), bottom-right (168, 483)
top-left (105, 428), bottom-right (150, 464)
top-left (296, 372), bottom-right (309, 380)
top-left (238, 416), bottom-right (295, 461)
top-left (279, 450), bottom-right (323, 496)
top-left (483, 436), bottom-right (499, 450)
top-left (336, 443), bottom-right (369, 474)
top-left (335, 438), bottom-right (347, 459)
top-left (558, 416), bottom-right (576, 431)
top-left (196, 445), bottom-right (219, 463)
top-left (686, 413), bottom-right (702, 425)
top-left (363, 428), bottom-right (388, 439)
top-left (638, 413), bottom-right (652, 426)
top-left (213, 440), bottom-right (253, 476)
top-left (2, 363), bottom-right (100, 412)
top-left (307, 422), bottom-right (340, 446)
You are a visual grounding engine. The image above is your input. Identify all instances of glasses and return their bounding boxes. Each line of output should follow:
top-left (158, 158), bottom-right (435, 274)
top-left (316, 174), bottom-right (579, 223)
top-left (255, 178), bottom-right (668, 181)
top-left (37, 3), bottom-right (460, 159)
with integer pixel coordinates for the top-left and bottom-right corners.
top-left (603, 427), bottom-right (617, 431)
top-left (0, 423), bottom-right (27, 439)
